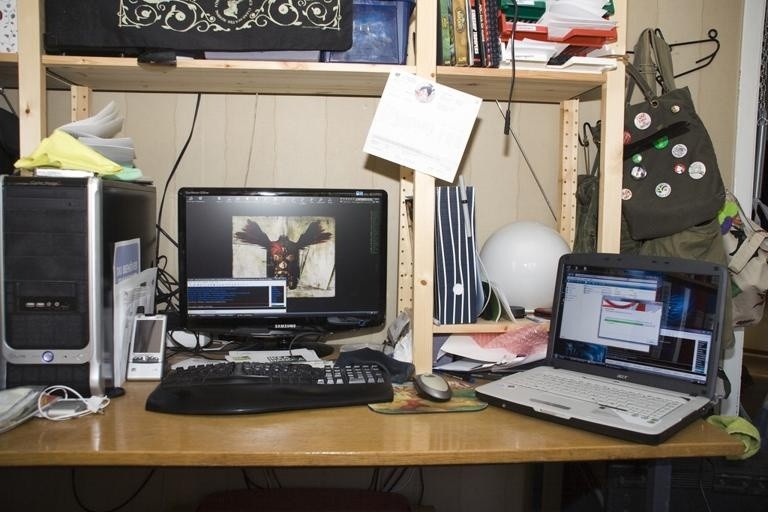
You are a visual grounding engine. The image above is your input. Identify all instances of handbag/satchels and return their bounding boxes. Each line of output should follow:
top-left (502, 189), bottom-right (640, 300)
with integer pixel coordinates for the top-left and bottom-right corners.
top-left (573, 175), bottom-right (640, 257)
top-left (404, 185), bottom-right (479, 325)
top-left (636, 216), bottom-right (736, 349)
top-left (718, 187), bottom-right (768, 329)
top-left (590, 62), bottom-right (726, 241)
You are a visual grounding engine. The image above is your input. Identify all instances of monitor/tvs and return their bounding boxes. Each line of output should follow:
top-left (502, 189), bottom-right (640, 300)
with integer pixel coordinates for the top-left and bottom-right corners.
top-left (178, 188), bottom-right (388, 358)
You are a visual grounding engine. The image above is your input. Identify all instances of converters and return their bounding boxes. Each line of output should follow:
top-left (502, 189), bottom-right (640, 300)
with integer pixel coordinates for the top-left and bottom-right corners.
top-left (157, 309), bottom-right (184, 331)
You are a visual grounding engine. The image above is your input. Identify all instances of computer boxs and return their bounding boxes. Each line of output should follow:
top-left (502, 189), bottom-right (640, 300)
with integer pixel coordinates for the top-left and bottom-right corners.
top-left (0, 174), bottom-right (157, 397)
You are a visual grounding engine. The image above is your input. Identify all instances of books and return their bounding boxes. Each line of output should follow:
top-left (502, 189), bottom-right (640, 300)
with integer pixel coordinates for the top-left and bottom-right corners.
top-left (438, 0), bottom-right (500, 68)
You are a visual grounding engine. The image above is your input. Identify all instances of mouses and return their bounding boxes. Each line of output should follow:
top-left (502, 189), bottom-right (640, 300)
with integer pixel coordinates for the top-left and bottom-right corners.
top-left (413, 373), bottom-right (452, 402)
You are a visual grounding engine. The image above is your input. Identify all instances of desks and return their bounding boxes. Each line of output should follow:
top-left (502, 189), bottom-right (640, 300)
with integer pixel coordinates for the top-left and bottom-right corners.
top-left (1, 348), bottom-right (743, 512)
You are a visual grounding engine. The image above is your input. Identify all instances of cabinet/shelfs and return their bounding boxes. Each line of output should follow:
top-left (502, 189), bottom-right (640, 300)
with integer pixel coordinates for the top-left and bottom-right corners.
top-left (0, 0), bottom-right (627, 375)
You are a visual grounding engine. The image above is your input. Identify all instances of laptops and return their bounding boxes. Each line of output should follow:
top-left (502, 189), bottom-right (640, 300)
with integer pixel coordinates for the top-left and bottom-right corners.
top-left (475, 252), bottom-right (728, 446)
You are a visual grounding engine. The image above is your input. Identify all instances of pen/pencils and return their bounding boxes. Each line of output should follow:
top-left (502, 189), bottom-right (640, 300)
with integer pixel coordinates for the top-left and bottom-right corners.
top-left (443, 370), bottom-right (476, 384)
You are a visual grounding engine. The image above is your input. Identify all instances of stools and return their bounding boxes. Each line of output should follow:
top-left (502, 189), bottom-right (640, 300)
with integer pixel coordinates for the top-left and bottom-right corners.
top-left (195, 488), bottom-right (410, 512)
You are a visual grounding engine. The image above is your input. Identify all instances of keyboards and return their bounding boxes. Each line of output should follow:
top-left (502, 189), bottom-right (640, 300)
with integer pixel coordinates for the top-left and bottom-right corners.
top-left (146, 361), bottom-right (394, 416)
